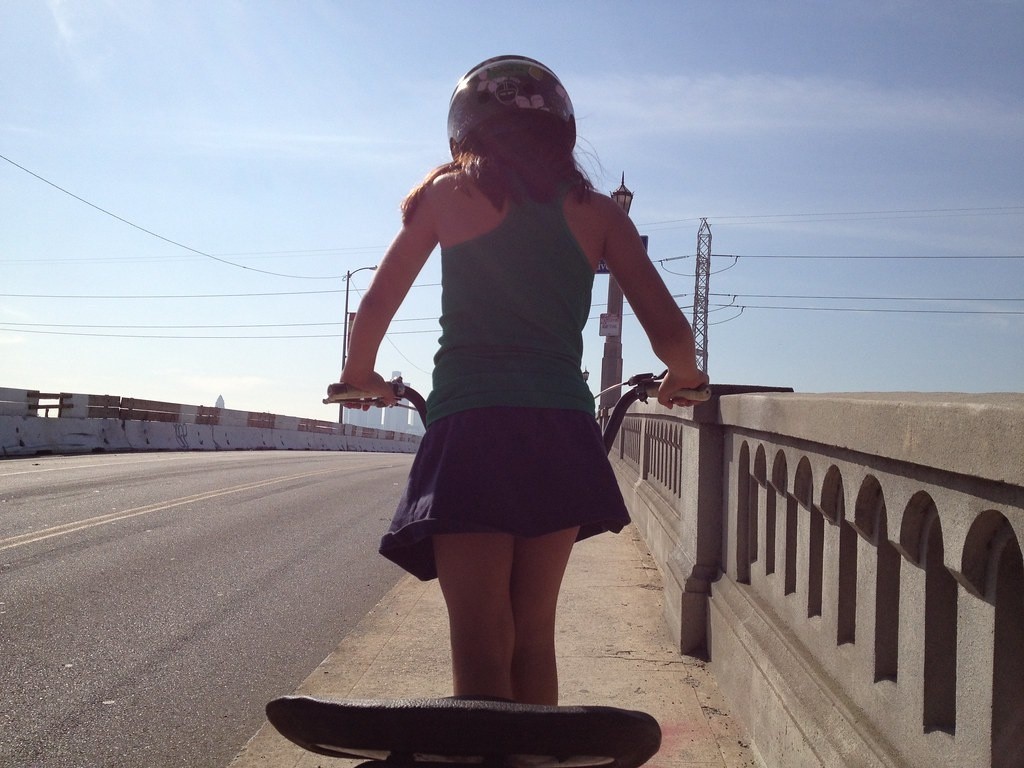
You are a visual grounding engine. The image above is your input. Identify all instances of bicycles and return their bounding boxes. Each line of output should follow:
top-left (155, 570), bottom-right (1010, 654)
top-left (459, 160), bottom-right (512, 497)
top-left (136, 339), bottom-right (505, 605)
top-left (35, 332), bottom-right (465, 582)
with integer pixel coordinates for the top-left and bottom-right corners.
top-left (266, 371), bottom-right (714, 768)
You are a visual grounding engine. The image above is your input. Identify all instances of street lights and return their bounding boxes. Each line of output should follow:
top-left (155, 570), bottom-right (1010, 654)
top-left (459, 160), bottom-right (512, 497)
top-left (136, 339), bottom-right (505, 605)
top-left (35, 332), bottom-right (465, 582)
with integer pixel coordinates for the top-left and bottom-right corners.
top-left (337, 264), bottom-right (376, 425)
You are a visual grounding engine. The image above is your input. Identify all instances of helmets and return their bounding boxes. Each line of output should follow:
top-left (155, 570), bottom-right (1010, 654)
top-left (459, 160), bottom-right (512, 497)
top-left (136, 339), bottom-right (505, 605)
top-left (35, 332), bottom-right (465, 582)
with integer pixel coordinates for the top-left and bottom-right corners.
top-left (447, 55), bottom-right (576, 153)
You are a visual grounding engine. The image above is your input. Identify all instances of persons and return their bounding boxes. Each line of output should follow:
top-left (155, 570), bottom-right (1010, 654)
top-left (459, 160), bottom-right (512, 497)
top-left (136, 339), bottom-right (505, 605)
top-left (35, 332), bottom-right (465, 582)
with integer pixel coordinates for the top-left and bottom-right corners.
top-left (340, 54), bottom-right (709, 706)
